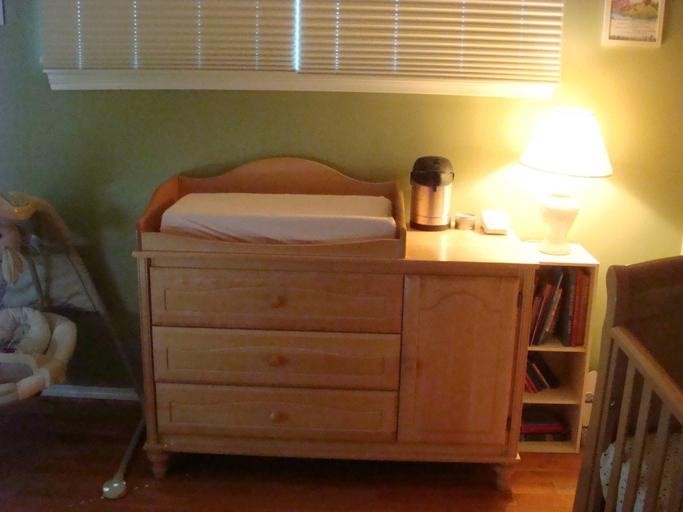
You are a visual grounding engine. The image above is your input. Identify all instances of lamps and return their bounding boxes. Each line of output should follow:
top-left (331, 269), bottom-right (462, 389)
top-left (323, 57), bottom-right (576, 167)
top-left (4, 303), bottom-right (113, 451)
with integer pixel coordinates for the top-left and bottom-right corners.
top-left (514, 87), bottom-right (613, 255)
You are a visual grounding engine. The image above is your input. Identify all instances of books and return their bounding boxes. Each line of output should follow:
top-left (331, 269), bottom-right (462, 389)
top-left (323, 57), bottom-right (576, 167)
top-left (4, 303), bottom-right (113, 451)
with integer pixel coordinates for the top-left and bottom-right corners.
top-left (518, 265), bottom-right (589, 443)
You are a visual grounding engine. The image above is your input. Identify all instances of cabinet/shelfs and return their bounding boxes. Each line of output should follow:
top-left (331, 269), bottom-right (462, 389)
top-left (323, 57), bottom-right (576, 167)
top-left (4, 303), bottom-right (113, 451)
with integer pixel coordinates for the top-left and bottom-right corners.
top-left (519, 241), bottom-right (597, 456)
top-left (132, 152), bottom-right (539, 496)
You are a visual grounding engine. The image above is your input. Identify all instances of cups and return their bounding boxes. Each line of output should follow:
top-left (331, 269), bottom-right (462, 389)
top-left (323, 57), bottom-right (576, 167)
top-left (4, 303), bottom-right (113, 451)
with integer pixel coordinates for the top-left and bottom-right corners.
top-left (455, 214), bottom-right (475, 230)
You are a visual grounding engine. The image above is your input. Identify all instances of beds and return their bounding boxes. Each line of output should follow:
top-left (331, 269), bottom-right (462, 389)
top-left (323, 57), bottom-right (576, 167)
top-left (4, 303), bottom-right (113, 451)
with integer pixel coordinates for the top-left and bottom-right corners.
top-left (569, 253), bottom-right (682, 512)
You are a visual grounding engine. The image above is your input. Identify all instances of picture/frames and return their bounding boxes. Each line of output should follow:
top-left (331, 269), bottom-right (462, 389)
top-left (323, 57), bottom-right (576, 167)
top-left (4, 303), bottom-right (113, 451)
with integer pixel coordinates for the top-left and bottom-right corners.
top-left (601, 1), bottom-right (668, 52)
